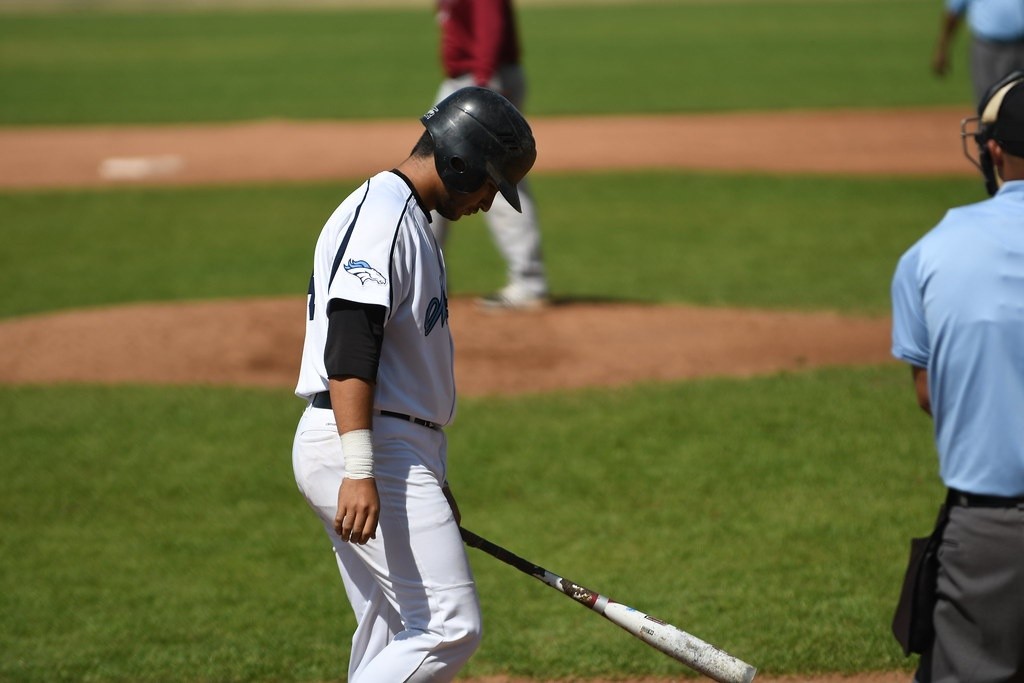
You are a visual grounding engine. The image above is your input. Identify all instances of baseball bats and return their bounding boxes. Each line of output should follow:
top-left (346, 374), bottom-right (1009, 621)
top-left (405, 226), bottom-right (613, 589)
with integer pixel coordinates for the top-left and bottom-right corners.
top-left (459, 526), bottom-right (757, 683)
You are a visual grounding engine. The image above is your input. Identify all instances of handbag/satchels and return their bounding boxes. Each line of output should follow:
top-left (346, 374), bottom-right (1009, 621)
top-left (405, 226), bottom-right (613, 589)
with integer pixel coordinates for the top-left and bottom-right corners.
top-left (890, 534), bottom-right (940, 657)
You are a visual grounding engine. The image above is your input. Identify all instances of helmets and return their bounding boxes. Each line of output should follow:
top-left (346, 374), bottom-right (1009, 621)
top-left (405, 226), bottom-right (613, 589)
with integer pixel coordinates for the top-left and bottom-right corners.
top-left (418, 85), bottom-right (538, 215)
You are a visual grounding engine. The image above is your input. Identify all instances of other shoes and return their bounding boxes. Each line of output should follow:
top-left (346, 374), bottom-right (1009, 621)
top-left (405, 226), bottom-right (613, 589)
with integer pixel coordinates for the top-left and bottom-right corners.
top-left (468, 284), bottom-right (551, 315)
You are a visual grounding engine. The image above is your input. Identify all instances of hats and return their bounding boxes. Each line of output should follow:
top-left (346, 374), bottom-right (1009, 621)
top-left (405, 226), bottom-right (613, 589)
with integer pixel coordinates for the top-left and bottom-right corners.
top-left (979, 75), bottom-right (1024, 142)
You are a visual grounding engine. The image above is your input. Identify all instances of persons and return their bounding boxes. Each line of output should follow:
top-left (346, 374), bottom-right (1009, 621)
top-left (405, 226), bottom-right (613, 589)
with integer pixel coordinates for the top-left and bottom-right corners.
top-left (290, 86), bottom-right (541, 683)
top-left (886, 70), bottom-right (1024, 683)
top-left (426, 0), bottom-right (552, 308)
top-left (931, 0), bottom-right (1023, 125)
